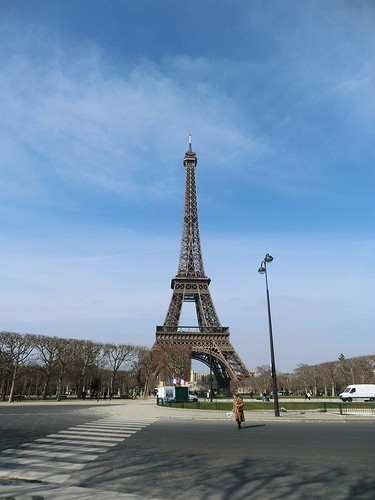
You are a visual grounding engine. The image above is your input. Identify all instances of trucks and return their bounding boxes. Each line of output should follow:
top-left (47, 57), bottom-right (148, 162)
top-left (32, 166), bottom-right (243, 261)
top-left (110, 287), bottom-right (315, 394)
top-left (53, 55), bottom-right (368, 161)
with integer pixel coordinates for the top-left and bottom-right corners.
top-left (156, 385), bottom-right (198, 403)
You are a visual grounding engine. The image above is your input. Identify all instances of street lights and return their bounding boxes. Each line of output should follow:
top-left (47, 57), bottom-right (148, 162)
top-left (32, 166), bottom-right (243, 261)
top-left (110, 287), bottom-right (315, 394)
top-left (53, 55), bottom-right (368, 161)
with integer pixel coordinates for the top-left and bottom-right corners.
top-left (258, 253), bottom-right (281, 417)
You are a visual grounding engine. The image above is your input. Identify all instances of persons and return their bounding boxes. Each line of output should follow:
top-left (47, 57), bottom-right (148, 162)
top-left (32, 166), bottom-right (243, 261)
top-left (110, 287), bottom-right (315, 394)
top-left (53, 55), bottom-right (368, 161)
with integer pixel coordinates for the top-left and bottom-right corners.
top-left (207, 390), bottom-right (210, 401)
top-left (304, 392), bottom-right (311, 400)
top-left (81, 390), bottom-right (113, 402)
top-left (250, 390), bottom-right (253, 399)
top-left (262, 391), bottom-right (265, 401)
top-left (233, 393), bottom-right (245, 430)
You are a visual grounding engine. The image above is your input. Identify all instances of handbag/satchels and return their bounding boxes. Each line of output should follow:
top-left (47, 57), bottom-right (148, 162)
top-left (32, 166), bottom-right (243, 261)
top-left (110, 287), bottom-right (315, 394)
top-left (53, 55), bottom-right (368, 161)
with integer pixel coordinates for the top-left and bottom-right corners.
top-left (226, 412), bottom-right (233, 418)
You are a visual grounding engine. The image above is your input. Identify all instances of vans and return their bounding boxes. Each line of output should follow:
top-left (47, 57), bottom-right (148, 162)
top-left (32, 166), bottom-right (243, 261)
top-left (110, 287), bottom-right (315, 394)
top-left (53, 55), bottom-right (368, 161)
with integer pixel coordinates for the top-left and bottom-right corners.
top-left (339, 383), bottom-right (375, 402)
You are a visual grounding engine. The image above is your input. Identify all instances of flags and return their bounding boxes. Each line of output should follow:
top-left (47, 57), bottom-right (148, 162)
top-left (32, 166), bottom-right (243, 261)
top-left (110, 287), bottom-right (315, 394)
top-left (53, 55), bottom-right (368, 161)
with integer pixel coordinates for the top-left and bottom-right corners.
top-left (170, 378), bottom-right (188, 385)
